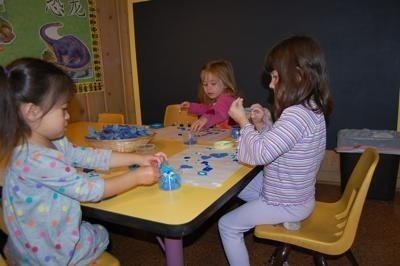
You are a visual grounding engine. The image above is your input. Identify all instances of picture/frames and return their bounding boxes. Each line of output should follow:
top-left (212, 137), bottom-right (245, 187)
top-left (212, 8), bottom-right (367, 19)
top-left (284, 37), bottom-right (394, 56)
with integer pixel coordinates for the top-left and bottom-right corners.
top-left (0, 0), bottom-right (105, 94)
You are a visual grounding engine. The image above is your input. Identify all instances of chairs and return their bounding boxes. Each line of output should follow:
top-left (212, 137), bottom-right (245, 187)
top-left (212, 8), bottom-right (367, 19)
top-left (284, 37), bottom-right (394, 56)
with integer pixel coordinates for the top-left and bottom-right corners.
top-left (98, 113), bottom-right (124, 124)
top-left (0, 239), bottom-right (121, 266)
top-left (164, 104), bottom-right (199, 128)
top-left (253, 148), bottom-right (380, 266)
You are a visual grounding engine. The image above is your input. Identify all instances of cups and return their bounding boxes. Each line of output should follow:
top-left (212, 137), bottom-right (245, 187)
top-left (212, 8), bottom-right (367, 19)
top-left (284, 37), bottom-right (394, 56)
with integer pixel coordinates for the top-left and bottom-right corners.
top-left (136, 144), bottom-right (155, 155)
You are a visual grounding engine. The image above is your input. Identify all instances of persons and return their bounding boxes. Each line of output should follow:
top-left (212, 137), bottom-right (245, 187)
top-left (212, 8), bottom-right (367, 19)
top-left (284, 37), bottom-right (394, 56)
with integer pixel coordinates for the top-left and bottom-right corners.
top-left (216, 33), bottom-right (333, 265)
top-left (180, 60), bottom-right (241, 135)
top-left (0, 56), bottom-right (170, 266)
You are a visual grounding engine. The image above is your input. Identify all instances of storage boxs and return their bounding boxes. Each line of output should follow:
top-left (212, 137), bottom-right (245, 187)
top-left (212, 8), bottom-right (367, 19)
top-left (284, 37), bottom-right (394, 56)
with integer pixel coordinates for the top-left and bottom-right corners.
top-left (335, 130), bottom-right (400, 200)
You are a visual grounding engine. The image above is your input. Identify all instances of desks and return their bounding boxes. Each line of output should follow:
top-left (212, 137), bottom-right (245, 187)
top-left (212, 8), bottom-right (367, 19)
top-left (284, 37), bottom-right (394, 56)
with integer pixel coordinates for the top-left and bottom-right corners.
top-left (0, 121), bottom-right (265, 266)
